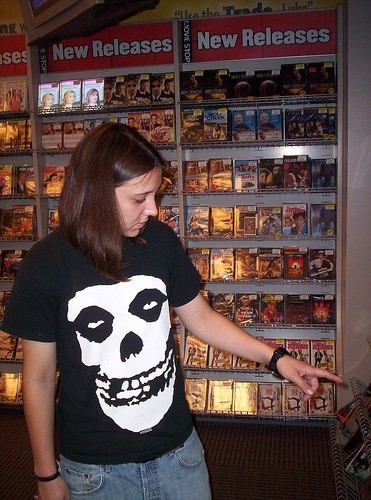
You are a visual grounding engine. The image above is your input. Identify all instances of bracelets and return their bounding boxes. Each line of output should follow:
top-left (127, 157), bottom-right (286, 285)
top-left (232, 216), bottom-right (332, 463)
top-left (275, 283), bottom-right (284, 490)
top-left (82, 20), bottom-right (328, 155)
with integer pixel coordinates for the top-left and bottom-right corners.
top-left (33, 472), bottom-right (60, 482)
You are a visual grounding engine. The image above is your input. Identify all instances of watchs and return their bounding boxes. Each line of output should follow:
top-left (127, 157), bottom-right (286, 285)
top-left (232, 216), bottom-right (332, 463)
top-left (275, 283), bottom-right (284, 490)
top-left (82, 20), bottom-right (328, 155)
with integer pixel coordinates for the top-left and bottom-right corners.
top-left (268, 347), bottom-right (290, 378)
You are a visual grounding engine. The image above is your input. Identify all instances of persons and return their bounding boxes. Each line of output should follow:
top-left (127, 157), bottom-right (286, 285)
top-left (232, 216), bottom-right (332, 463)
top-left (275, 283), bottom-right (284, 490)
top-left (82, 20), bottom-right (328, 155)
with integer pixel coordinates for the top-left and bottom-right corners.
top-left (0, 64), bottom-right (338, 412)
top-left (0, 122), bottom-right (346, 500)
